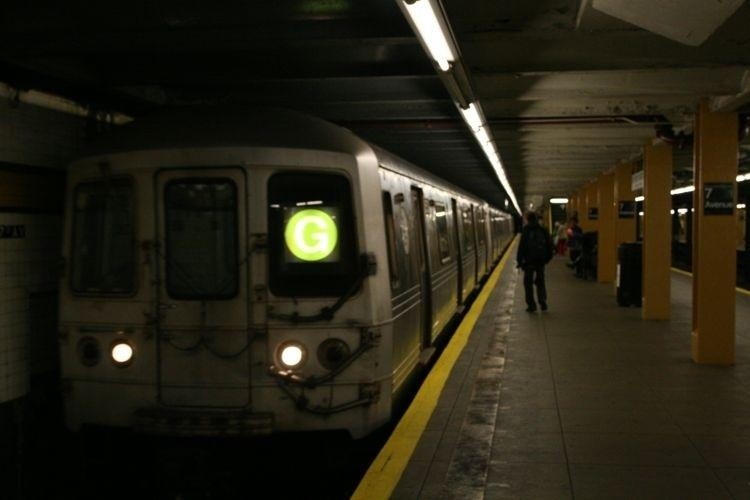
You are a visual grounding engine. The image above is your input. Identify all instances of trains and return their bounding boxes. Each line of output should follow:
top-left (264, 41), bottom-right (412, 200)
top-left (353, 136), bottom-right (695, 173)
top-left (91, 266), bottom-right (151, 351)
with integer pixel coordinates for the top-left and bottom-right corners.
top-left (57, 103), bottom-right (515, 440)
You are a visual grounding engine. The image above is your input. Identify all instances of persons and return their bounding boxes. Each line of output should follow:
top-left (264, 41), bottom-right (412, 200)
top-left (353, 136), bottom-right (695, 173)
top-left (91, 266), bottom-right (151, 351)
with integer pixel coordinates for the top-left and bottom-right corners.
top-left (518, 211), bottom-right (551, 313)
top-left (539, 216), bottom-right (589, 268)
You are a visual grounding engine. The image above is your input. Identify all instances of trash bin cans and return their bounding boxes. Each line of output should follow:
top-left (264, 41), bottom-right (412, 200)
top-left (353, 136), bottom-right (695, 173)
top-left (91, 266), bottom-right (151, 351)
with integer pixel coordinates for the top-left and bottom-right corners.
top-left (616, 241), bottom-right (642, 307)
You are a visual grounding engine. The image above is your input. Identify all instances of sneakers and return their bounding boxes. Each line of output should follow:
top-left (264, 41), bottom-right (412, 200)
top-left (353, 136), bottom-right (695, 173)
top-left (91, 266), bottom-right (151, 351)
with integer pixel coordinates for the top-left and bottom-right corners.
top-left (526, 302), bottom-right (549, 313)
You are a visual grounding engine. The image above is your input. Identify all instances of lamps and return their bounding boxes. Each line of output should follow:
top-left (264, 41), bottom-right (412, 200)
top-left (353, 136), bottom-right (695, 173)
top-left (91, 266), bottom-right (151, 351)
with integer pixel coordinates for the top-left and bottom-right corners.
top-left (398, 0), bottom-right (525, 217)
